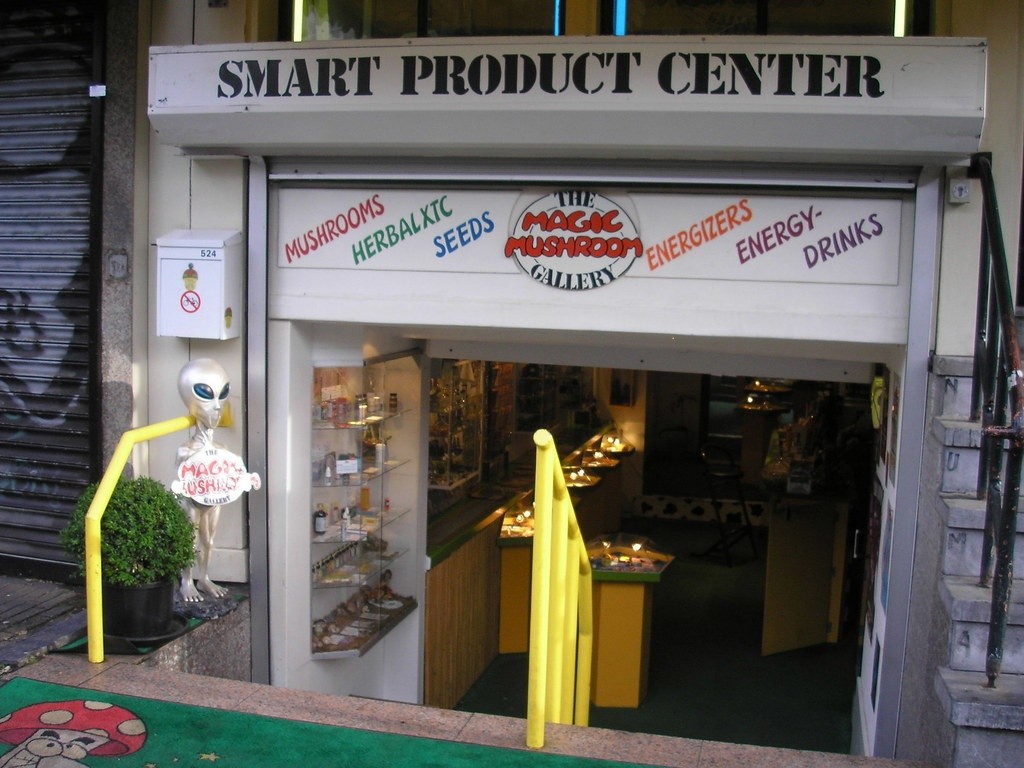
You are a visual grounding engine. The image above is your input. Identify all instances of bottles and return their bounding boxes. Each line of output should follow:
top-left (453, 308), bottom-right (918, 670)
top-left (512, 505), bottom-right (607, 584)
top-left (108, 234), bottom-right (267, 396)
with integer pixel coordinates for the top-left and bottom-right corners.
top-left (314, 503), bottom-right (327, 534)
top-left (779, 423), bottom-right (803, 459)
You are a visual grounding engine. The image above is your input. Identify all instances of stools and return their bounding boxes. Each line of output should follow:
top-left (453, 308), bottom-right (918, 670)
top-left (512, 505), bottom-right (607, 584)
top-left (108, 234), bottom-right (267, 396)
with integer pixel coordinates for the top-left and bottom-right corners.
top-left (690, 444), bottom-right (758, 567)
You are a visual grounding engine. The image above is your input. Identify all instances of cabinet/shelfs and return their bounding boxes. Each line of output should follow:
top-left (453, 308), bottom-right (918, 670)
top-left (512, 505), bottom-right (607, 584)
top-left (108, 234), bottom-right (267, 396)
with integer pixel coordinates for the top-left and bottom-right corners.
top-left (565, 473), bottom-right (608, 541)
top-left (760, 487), bottom-right (849, 657)
top-left (428, 360), bottom-right (484, 519)
top-left (495, 503), bottom-right (533, 657)
top-left (598, 443), bottom-right (638, 477)
top-left (590, 546), bottom-right (677, 709)
top-left (513, 364), bottom-right (561, 436)
top-left (312, 405), bottom-right (419, 660)
top-left (582, 457), bottom-right (624, 533)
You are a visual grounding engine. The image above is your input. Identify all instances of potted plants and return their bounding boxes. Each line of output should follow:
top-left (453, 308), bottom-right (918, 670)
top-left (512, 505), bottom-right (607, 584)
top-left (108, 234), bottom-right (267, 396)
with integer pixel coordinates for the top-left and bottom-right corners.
top-left (59, 475), bottom-right (201, 637)
